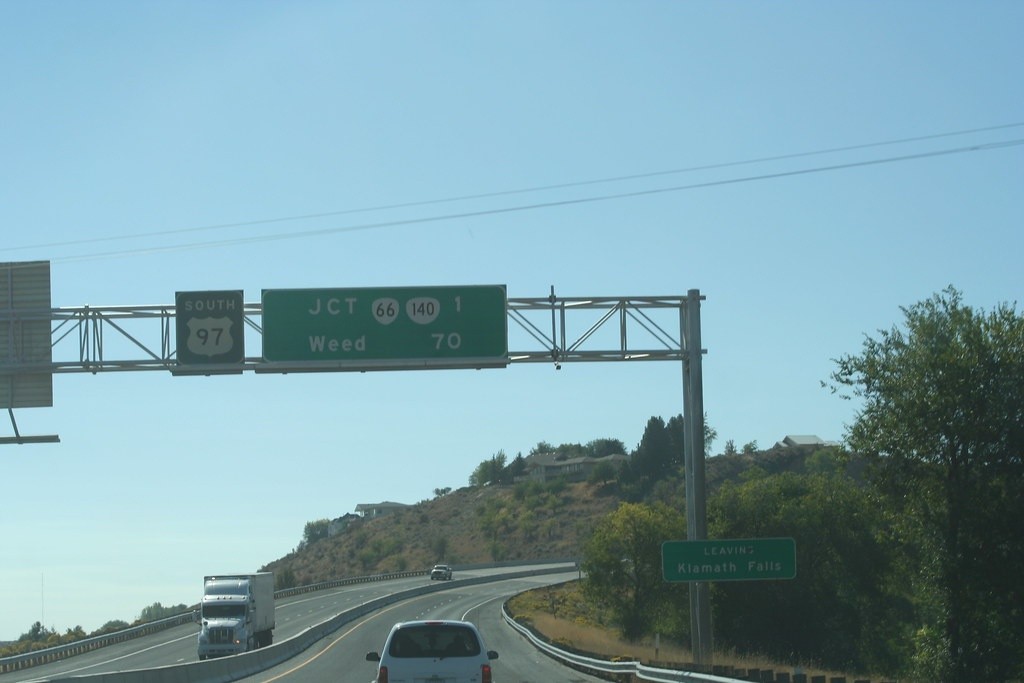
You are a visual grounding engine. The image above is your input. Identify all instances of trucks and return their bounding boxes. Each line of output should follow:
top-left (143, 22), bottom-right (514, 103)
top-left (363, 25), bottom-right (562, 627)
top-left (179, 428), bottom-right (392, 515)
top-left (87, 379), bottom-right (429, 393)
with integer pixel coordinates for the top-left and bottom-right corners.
top-left (191, 571), bottom-right (276, 660)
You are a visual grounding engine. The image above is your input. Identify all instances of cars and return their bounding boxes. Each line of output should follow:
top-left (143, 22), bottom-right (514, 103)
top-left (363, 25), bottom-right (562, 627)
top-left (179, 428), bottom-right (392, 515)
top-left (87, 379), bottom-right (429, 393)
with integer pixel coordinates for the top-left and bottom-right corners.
top-left (430, 565), bottom-right (453, 581)
top-left (366, 620), bottom-right (499, 683)
